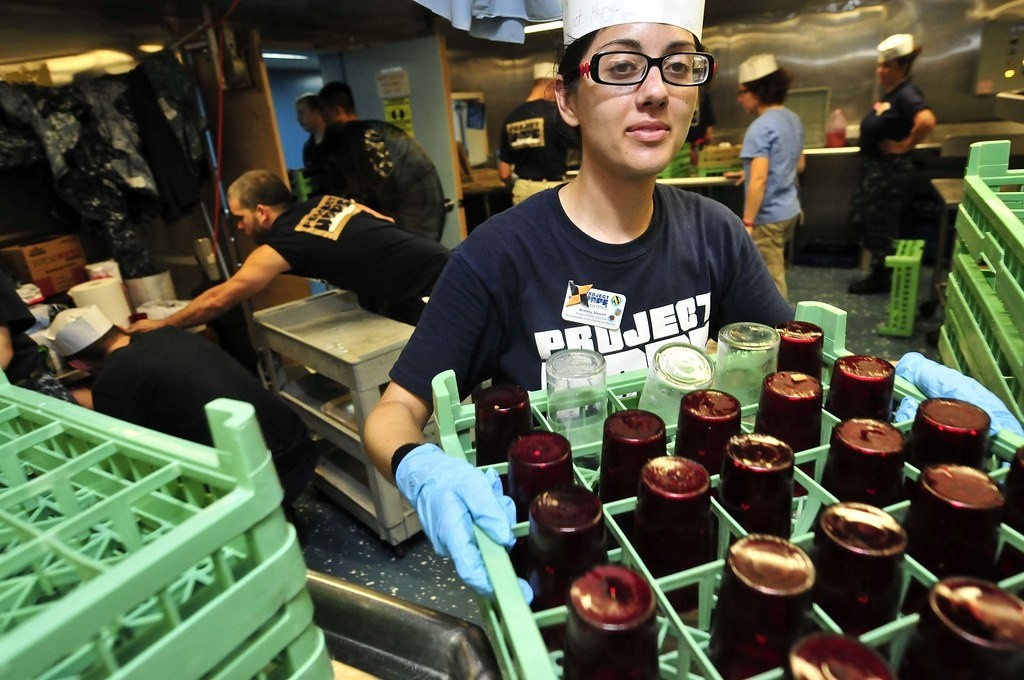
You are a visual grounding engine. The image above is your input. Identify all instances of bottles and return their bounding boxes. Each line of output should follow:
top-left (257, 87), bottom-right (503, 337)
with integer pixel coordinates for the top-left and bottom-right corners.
top-left (826, 109), bottom-right (847, 148)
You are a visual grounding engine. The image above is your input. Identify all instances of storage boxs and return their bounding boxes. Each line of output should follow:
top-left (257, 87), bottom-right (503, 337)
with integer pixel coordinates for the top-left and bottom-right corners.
top-left (0, 139), bottom-right (1024, 680)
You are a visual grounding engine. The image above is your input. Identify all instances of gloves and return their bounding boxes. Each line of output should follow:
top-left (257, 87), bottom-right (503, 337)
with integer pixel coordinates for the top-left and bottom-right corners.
top-left (892, 350), bottom-right (1023, 485)
top-left (393, 442), bottom-right (535, 607)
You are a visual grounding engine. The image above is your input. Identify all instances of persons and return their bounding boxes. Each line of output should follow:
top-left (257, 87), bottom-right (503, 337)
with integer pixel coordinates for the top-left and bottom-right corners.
top-left (499, 62), bottom-right (581, 206)
top-left (126, 169), bottom-right (452, 327)
top-left (0, 274), bottom-right (79, 405)
top-left (295, 81), bottom-right (445, 242)
top-left (846, 33), bottom-right (936, 295)
top-left (363, 0), bottom-right (1024, 605)
top-left (43, 306), bottom-right (320, 506)
top-left (725, 53), bottom-right (806, 304)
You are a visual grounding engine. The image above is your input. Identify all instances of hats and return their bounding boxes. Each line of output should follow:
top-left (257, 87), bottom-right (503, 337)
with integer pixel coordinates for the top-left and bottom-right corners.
top-left (42, 304), bottom-right (114, 358)
top-left (533, 61), bottom-right (559, 80)
top-left (876, 33), bottom-right (914, 64)
top-left (737, 53), bottom-right (778, 84)
top-left (563, 0), bottom-right (707, 52)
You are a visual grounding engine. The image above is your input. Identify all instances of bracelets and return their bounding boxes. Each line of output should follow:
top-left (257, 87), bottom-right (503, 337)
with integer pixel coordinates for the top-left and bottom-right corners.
top-left (742, 218), bottom-right (755, 227)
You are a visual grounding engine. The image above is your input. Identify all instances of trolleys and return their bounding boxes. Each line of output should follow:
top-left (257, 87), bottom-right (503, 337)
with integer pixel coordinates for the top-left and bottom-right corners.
top-left (251, 287), bottom-right (441, 558)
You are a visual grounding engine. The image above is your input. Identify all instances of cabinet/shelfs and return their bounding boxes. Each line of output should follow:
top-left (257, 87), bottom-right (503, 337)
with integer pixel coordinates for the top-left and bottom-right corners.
top-left (250, 287), bottom-right (439, 558)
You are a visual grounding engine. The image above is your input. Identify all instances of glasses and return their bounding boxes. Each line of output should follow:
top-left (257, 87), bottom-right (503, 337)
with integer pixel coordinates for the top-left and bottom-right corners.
top-left (563, 49), bottom-right (716, 87)
top-left (737, 88), bottom-right (749, 96)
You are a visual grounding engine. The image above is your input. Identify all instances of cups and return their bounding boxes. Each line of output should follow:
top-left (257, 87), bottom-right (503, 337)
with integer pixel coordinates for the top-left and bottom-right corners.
top-left (675, 388), bottom-right (742, 475)
top-left (475, 385), bottom-right (532, 467)
top-left (902, 464), bottom-right (1007, 613)
top-left (526, 487), bottom-right (609, 648)
top-left (906, 396), bottom-right (990, 498)
top-left (713, 323), bottom-right (779, 423)
top-left (601, 410), bottom-right (667, 502)
top-left (813, 503), bottom-right (908, 661)
top-left (897, 578), bottom-right (1024, 680)
top-left (822, 417), bottom-right (908, 510)
top-left (823, 355), bottom-right (895, 422)
top-left (636, 343), bottom-right (715, 427)
top-left (634, 456), bottom-right (710, 620)
top-left (562, 566), bottom-right (658, 680)
top-left (719, 434), bottom-right (795, 544)
top-left (775, 322), bottom-right (824, 379)
top-left (1009, 446), bottom-right (1024, 533)
top-left (753, 372), bottom-right (822, 496)
top-left (507, 431), bottom-right (573, 524)
top-left (708, 535), bottom-right (818, 680)
top-left (782, 632), bottom-right (896, 680)
top-left (545, 348), bottom-right (607, 445)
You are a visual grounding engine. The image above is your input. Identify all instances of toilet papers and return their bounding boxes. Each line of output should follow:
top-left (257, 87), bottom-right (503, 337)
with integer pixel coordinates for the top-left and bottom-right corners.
top-left (66, 277), bottom-right (132, 331)
top-left (123, 268), bottom-right (177, 312)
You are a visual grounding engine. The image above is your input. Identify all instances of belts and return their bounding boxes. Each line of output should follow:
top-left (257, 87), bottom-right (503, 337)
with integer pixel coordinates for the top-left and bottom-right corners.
top-left (517, 175), bottom-right (563, 181)
top-left (11, 367), bottom-right (41, 386)
